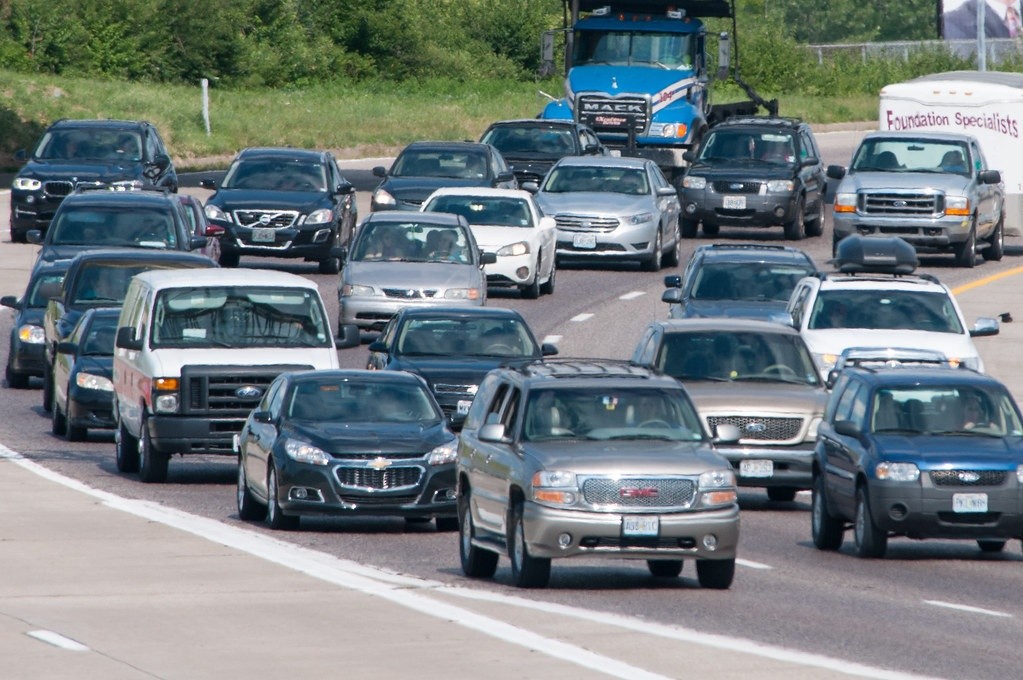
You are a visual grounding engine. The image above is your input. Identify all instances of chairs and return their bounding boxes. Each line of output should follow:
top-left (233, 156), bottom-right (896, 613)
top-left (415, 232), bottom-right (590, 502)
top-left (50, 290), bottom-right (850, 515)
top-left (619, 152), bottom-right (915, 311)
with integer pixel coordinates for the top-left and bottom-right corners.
top-left (416, 327), bottom-right (443, 354)
top-left (711, 336), bottom-right (747, 374)
top-left (571, 171), bottom-right (592, 189)
top-left (360, 393), bottom-right (381, 419)
top-left (413, 159), bottom-right (440, 175)
top-left (507, 134), bottom-right (531, 149)
top-left (247, 163), bottom-right (275, 181)
top-left (447, 205), bottom-right (474, 221)
top-left (748, 341), bottom-right (781, 375)
top-left (722, 137), bottom-right (749, 157)
top-left (870, 152), bottom-right (899, 167)
top-left (903, 399), bottom-right (929, 431)
top-left (439, 330), bottom-right (467, 351)
top-left (664, 338), bottom-right (691, 374)
top-left (528, 389), bottom-right (565, 436)
top-left (873, 393), bottom-right (898, 430)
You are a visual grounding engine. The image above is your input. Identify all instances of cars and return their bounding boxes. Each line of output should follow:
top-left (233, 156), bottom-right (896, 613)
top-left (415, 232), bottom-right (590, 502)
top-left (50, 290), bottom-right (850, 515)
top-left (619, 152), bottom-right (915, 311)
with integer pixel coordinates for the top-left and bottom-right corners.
top-left (520, 153), bottom-right (682, 274)
top-left (0, 256), bottom-right (77, 389)
top-left (329, 208), bottom-right (498, 339)
top-left (363, 304), bottom-right (560, 435)
top-left (48, 308), bottom-right (124, 442)
top-left (181, 194), bottom-right (227, 264)
top-left (369, 141), bottom-right (519, 213)
top-left (659, 241), bottom-right (818, 327)
top-left (398, 184), bottom-right (558, 300)
top-left (233, 369), bottom-right (468, 533)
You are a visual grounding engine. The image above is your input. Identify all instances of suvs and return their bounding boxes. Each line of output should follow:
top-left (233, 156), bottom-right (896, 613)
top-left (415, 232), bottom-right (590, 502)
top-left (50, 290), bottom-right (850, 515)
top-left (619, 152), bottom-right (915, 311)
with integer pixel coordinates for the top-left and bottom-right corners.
top-left (464, 118), bottom-right (612, 196)
top-left (9, 117), bottom-right (180, 243)
top-left (784, 232), bottom-right (1001, 383)
top-left (198, 145), bottom-right (359, 274)
top-left (34, 248), bottom-right (225, 414)
top-left (825, 126), bottom-right (1008, 269)
top-left (807, 372), bottom-right (1022, 560)
top-left (677, 115), bottom-right (829, 240)
top-left (26, 182), bottom-right (208, 271)
top-left (627, 318), bottom-right (832, 501)
top-left (451, 356), bottom-right (740, 589)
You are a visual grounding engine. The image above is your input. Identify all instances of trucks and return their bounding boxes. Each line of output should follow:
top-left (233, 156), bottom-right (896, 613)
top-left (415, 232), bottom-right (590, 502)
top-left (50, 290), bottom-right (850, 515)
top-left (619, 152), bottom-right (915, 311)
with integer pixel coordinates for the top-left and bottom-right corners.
top-left (533, 0), bottom-right (780, 183)
top-left (876, 67), bottom-right (1021, 239)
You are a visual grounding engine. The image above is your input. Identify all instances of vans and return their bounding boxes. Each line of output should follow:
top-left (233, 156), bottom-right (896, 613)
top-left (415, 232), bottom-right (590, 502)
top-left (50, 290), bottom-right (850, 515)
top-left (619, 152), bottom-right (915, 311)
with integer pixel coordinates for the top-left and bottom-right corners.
top-left (112, 268), bottom-right (361, 485)
